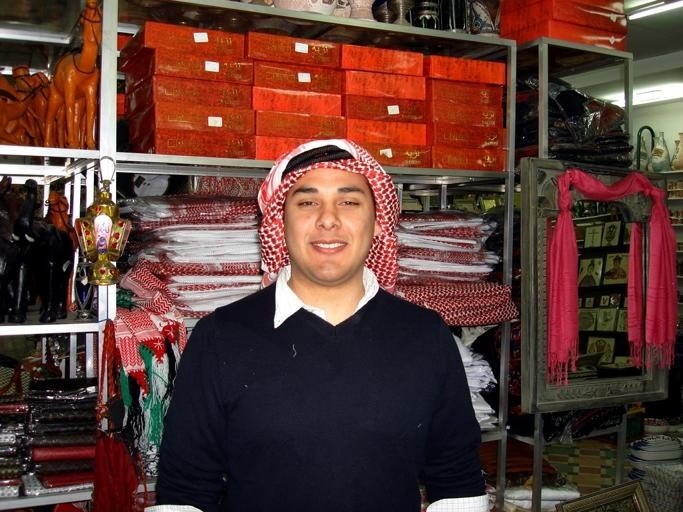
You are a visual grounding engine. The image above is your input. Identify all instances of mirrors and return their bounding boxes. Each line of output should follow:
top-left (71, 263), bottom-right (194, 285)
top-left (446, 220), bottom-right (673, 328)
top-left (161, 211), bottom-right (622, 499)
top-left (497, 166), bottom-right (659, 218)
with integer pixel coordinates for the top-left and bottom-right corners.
top-left (516, 155), bottom-right (679, 415)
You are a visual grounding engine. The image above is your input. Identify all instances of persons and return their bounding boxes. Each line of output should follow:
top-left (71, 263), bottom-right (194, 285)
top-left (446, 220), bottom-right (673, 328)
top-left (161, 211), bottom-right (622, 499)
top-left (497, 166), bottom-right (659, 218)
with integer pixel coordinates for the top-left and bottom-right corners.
top-left (578, 259), bottom-right (597, 287)
top-left (143, 137), bottom-right (492, 512)
top-left (604, 255), bottom-right (627, 279)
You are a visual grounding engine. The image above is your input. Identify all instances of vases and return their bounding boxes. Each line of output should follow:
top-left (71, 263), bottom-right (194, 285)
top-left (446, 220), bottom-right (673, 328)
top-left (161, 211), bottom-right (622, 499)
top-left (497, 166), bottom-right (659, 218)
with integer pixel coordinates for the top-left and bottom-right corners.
top-left (671, 133), bottom-right (682, 170)
top-left (649, 130), bottom-right (669, 171)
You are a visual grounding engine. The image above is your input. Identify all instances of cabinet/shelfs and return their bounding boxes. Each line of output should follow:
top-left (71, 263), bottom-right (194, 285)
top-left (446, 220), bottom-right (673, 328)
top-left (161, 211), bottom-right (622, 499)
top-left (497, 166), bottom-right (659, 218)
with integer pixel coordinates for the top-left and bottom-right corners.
top-left (469, 34), bottom-right (638, 512)
top-left (651, 169), bottom-right (682, 348)
top-left (0, 0), bottom-right (515, 512)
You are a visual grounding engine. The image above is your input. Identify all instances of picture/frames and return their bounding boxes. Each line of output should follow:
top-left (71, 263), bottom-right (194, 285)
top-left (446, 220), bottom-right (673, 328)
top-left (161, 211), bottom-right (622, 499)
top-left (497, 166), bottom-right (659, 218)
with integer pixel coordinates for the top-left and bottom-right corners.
top-left (554, 481), bottom-right (652, 512)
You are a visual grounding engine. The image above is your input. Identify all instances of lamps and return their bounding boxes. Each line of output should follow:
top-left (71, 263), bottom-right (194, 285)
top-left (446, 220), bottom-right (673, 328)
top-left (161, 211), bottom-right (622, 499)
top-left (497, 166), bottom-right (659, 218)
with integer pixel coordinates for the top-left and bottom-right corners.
top-left (74, 156), bottom-right (132, 287)
